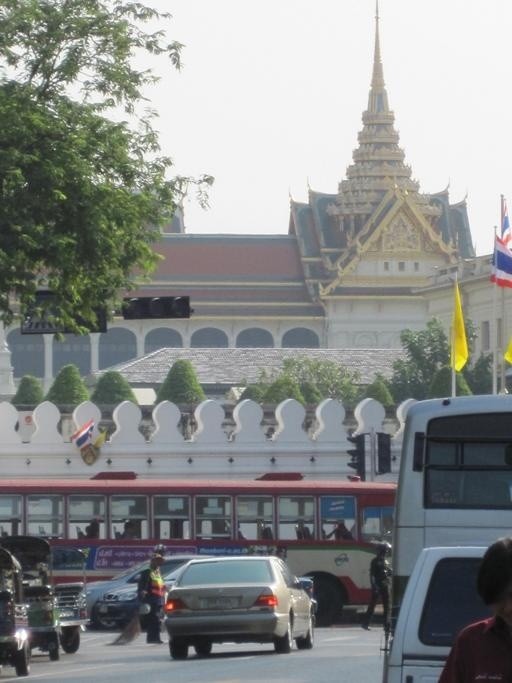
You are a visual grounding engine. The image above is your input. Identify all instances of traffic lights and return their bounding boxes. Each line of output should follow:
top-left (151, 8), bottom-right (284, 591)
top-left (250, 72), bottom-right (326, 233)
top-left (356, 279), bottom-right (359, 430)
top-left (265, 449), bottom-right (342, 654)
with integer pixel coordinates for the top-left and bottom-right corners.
top-left (120, 296), bottom-right (192, 320)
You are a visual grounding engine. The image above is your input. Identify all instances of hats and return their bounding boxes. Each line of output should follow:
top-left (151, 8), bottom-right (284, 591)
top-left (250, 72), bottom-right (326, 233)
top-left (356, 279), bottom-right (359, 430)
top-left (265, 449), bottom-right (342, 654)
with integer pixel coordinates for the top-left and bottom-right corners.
top-left (151, 553), bottom-right (165, 561)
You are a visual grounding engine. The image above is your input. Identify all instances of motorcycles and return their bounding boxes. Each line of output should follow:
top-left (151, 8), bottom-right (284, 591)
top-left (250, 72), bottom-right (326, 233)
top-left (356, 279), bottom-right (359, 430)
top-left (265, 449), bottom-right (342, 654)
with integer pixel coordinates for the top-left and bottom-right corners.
top-left (1, 534), bottom-right (86, 678)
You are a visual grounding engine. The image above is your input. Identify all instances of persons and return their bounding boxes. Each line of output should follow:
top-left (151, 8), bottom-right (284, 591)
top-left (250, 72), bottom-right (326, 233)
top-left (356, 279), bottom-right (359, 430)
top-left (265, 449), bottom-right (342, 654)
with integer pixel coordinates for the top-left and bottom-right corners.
top-left (361, 542), bottom-right (392, 630)
top-left (121, 522), bottom-right (138, 541)
top-left (437, 536), bottom-right (512, 683)
top-left (138, 554), bottom-right (172, 644)
top-left (430, 470), bottom-right (459, 506)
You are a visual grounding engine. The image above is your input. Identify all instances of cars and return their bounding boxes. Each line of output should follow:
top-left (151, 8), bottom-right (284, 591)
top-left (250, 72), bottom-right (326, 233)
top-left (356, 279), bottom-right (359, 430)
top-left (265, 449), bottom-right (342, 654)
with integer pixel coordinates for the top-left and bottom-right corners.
top-left (82, 555), bottom-right (315, 659)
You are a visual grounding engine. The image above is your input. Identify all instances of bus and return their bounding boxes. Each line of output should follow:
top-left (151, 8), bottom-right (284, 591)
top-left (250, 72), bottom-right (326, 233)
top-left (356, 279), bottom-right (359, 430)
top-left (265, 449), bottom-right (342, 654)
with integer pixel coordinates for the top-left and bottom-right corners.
top-left (0, 477), bottom-right (396, 628)
top-left (386, 396), bottom-right (512, 637)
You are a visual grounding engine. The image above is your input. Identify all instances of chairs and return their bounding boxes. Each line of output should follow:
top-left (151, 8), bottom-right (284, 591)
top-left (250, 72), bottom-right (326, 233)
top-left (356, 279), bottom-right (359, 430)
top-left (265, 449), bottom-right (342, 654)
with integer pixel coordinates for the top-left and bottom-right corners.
top-left (1, 520), bottom-right (354, 541)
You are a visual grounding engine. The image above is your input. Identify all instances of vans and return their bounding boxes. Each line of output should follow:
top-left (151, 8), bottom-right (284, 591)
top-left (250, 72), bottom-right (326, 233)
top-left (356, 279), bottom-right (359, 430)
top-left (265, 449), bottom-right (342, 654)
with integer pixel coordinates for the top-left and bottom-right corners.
top-left (381, 544), bottom-right (490, 683)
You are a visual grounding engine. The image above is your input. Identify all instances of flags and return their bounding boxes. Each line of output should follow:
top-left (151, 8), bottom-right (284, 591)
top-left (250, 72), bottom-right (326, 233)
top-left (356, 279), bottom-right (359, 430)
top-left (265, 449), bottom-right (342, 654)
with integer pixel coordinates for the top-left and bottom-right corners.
top-left (500, 198), bottom-right (512, 244)
top-left (449, 282), bottom-right (468, 373)
top-left (71, 419), bottom-right (94, 451)
top-left (490, 234), bottom-right (512, 287)
top-left (95, 430), bottom-right (107, 448)
